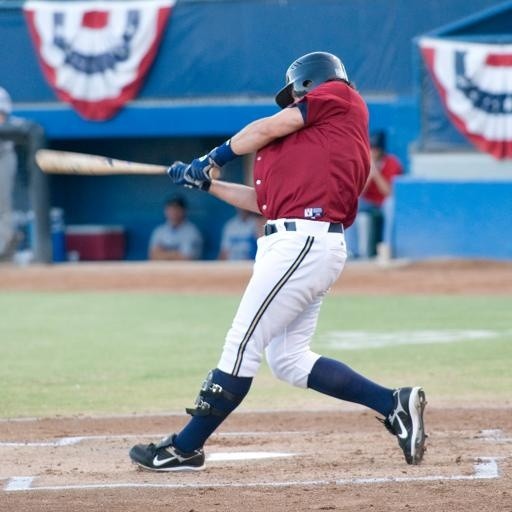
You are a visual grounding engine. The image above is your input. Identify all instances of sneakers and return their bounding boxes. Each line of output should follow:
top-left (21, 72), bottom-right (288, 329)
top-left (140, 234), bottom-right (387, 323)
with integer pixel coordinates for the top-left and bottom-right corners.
top-left (384, 385), bottom-right (427, 465)
top-left (129, 433), bottom-right (205, 471)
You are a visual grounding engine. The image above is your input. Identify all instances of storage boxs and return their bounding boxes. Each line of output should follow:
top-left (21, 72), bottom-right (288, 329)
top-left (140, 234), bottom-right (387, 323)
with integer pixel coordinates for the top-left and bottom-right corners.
top-left (67, 226), bottom-right (123, 260)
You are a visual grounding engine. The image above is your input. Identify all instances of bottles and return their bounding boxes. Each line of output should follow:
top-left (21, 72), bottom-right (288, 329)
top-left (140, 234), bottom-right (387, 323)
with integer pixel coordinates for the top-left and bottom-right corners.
top-left (49, 208), bottom-right (68, 263)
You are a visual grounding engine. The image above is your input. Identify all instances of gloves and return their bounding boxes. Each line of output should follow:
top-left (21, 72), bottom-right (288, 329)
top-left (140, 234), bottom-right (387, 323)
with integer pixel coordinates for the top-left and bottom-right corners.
top-left (166, 138), bottom-right (239, 192)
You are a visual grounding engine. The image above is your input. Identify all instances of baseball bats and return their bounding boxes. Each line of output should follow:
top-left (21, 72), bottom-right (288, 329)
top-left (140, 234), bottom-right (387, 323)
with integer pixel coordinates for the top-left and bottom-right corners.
top-left (37, 149), bottom-right (223, 181)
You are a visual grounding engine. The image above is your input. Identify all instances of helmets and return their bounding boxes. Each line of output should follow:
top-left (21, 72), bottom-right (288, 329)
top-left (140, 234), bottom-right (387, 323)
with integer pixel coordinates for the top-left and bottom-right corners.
top-left (275, 51), bottom-right (349, 109)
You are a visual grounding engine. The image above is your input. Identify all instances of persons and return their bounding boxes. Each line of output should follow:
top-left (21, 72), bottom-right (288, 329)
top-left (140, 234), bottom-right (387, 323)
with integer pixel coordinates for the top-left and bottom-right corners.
top-left (147, 189), bottom-right (204, 262)
top-left (358, 126), bottom-right (404, 260)
top-left (127, 49), bottom-right (431, 474)
top-left (217, 204), bottom-right (263, 260)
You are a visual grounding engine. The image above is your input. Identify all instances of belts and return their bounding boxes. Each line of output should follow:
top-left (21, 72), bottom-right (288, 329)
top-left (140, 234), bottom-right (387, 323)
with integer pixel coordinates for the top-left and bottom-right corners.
top-left (263, 222), bottom-right (343, 235)
